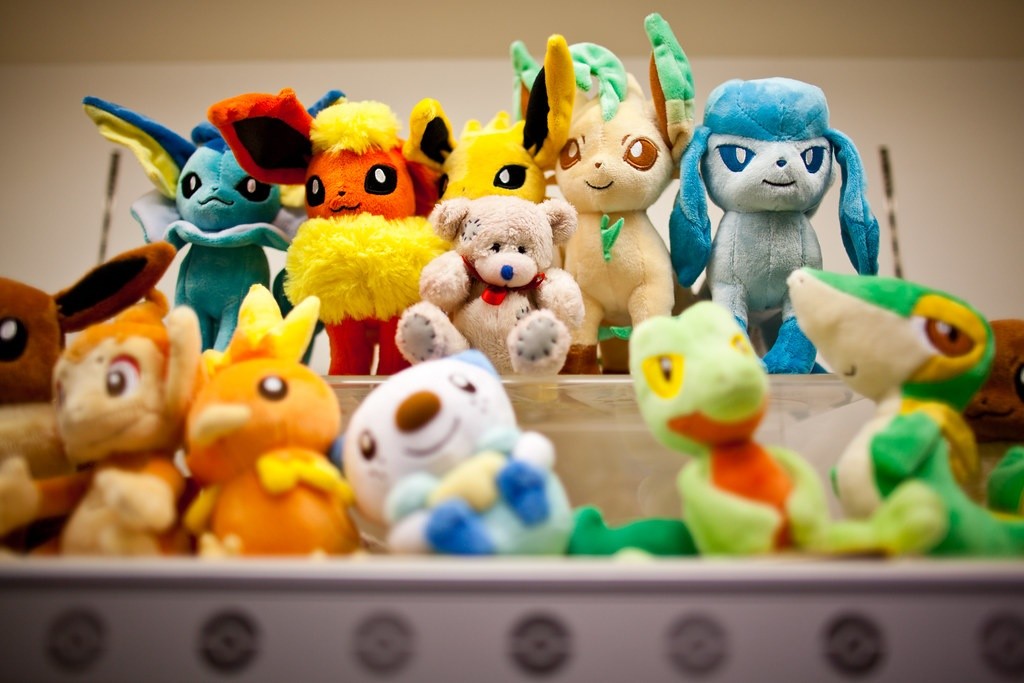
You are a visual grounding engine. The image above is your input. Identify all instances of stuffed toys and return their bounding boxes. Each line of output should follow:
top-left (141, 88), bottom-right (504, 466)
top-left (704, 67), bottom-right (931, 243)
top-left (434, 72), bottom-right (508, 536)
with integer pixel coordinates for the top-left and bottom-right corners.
top-left (0, 12), bottom-right (1024, 558)
